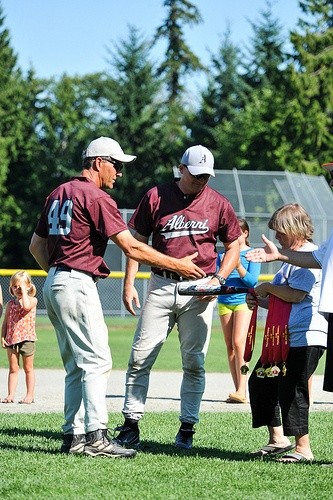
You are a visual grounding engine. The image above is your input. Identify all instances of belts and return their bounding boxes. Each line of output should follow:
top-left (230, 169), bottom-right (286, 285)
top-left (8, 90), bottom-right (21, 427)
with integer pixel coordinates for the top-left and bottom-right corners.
top-left (57, 266), bottom-right (98, 281)
top-left (151, 268), bottom-right (211, 281)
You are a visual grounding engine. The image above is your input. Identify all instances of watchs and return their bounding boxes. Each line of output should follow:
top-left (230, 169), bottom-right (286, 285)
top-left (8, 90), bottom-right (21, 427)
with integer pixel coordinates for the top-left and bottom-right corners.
top-left (214, 274), bottom-right (226, 285)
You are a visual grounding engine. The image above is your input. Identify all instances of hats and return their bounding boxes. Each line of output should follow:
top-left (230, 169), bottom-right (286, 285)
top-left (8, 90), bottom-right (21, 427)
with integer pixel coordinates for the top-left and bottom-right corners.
top-left (83, 136), bottom-right (137, 163)
top-left (181, 145), bottom-right (215, 177)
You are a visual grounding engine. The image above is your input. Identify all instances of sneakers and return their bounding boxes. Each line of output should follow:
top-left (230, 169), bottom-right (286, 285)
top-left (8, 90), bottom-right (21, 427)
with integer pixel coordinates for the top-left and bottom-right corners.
top-left (83, 428), bottom-right (136, 458)
top-left (112, 422), bottom-right (140, 451)
top-left (61, 434), bottom-right (86, 453)
top-left (175, 429), bottom-right (196, 450)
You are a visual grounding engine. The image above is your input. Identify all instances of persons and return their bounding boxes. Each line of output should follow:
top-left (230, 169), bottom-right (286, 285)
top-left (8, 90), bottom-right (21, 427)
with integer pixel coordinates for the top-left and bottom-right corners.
top-left (29, 136), bottom-right (206, 457)
top-left (0, 271), bottom-right (38, 404)
top-left (246, 163), bottom-right (333, 392)
top-left (112, 146), bottom-right (240, 450)
top-left (245, 203), bottom-right (328, 463)
top-left (215, 217), bottom-right (261, 404)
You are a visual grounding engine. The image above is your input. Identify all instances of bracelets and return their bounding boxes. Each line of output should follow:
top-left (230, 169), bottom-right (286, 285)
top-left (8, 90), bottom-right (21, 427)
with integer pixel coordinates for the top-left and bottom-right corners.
top-left (237, 265), bottom-right (242, 269)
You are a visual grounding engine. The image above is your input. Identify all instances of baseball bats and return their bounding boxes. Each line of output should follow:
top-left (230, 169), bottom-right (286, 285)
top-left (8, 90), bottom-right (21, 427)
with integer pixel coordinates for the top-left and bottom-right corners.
top-left (178, 285), bottom-right (252, 297)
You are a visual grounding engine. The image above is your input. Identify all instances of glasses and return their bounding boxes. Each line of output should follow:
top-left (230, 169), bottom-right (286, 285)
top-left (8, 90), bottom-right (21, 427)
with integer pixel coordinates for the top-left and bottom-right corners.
top-left (102, 158), bottom-right (124, 171)
top-left (186, 167), bottom-right (211, 179)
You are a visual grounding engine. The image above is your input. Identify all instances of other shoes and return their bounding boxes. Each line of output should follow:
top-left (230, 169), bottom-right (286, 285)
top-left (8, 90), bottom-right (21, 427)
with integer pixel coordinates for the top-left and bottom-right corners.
top-left (229, 393), bottom-right (246, 403)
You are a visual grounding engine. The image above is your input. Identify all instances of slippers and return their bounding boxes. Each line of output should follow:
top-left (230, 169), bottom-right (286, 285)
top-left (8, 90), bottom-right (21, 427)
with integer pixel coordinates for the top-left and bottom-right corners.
top-left (256, 443), bottom-right (297, 458)
top-left (276, 453), bottom-right (314, 462)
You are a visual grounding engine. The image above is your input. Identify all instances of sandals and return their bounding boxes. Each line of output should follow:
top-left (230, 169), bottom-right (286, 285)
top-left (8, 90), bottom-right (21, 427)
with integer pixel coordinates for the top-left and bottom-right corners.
top-left (1, 398), bottom-right (15, 403)
top-left (18, 397), bottom-right (35, 404)
